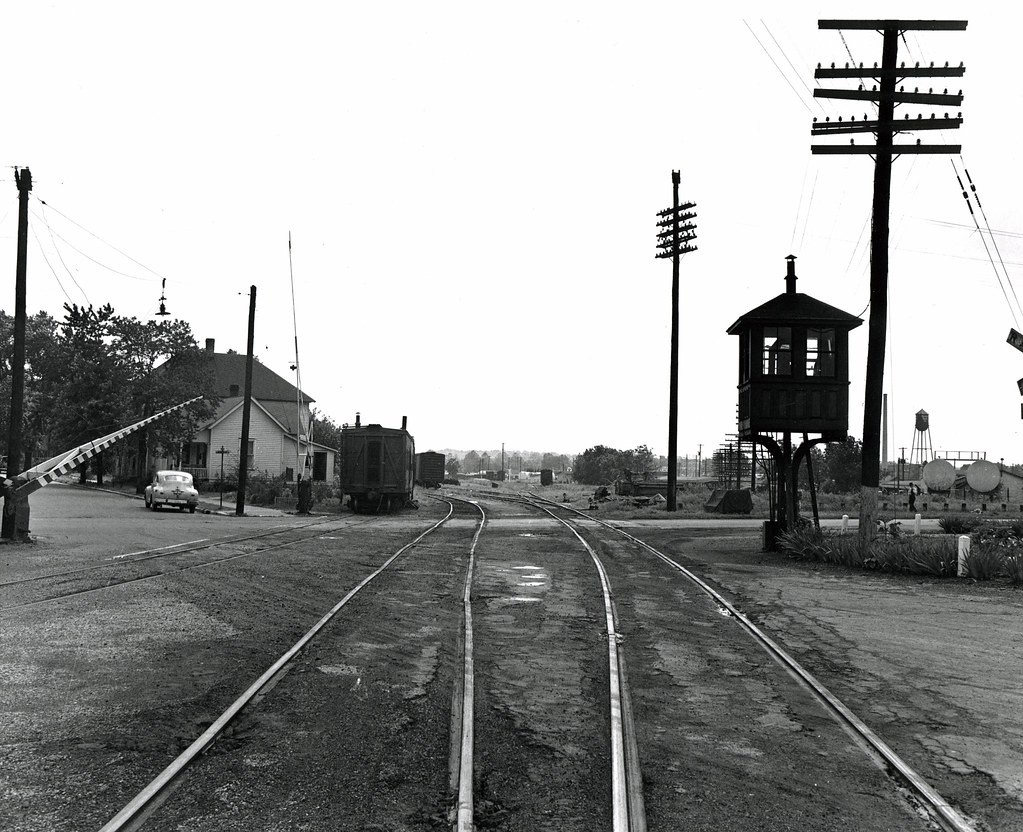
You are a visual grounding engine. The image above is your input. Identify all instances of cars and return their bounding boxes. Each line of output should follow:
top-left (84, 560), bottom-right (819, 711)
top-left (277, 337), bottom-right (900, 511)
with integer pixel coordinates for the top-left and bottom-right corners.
top-left (144, 470), bottom-right (199, 513)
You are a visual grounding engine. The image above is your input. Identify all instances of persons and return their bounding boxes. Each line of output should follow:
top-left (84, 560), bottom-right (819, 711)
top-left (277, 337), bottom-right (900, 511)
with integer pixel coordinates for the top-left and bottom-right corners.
top-left (908, 483), bottom-right (918, 512)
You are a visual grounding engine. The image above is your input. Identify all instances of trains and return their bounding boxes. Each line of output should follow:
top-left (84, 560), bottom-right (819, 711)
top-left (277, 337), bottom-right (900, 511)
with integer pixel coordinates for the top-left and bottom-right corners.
top-left (338, 415), bottom-right (418, 514)
top-left (413, 451), bottom-right (446, 489)
